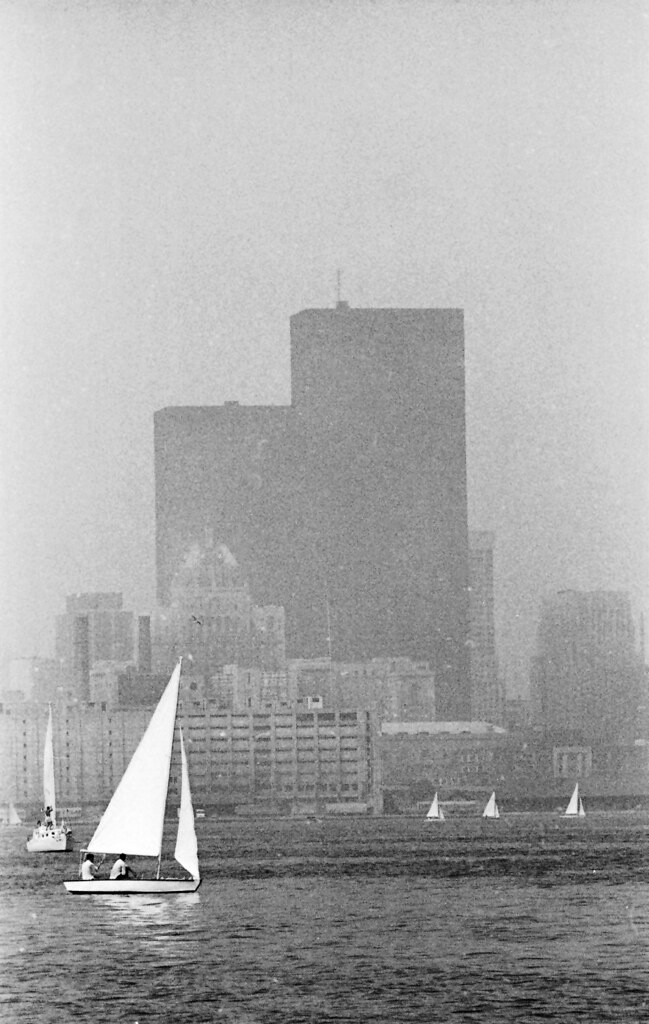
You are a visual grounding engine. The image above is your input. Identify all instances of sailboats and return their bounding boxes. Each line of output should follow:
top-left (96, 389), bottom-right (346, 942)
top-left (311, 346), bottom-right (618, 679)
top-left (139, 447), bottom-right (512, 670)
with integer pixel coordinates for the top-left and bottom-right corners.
top-left (557, 782), bottom-right (587, 819)
top-left (424, 790), bottom-right (447, 824)
top-left (480, 791), bottom-right (500, 822)
top-left (26, 700), bottom-right (76, 853)
top-left (61, 655), bottom-right (202, 893)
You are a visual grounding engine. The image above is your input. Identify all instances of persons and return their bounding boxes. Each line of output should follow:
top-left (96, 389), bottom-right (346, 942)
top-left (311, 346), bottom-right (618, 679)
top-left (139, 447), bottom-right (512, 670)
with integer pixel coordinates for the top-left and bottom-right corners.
top-left (36, 821), bottom-right (46, 832)
top-left (109, 853), bottom-right (135, 880)
top-left (82, 853), bottom-right (104, 880)
top-left (41, 806), bottom-right (53, 831)
